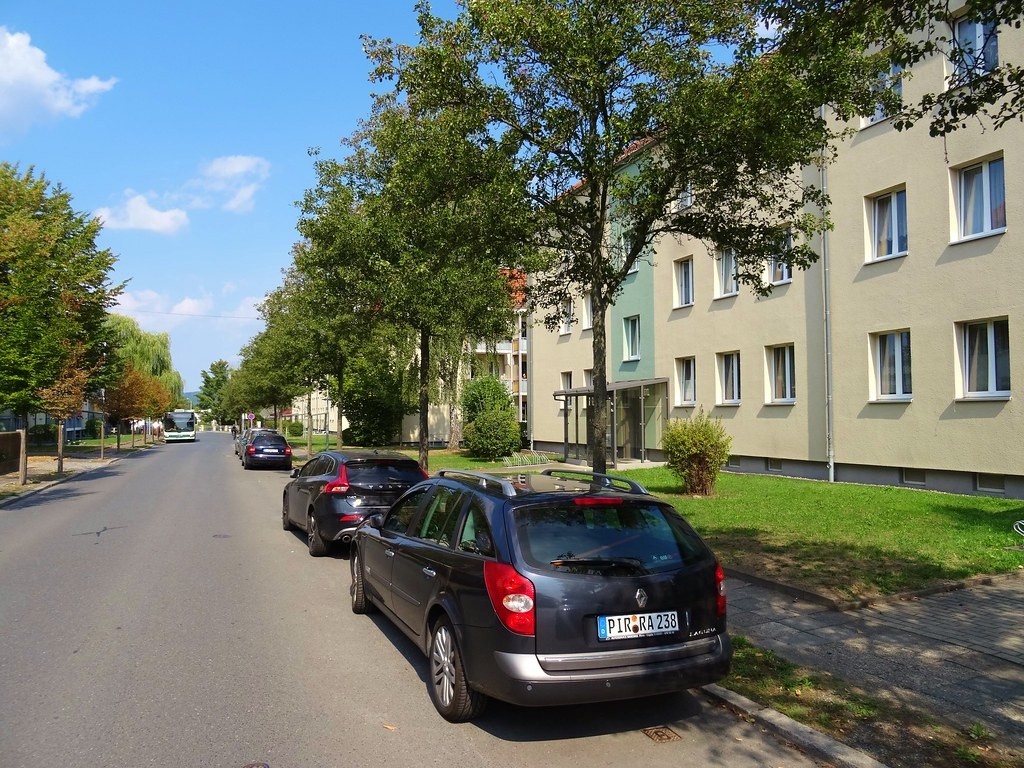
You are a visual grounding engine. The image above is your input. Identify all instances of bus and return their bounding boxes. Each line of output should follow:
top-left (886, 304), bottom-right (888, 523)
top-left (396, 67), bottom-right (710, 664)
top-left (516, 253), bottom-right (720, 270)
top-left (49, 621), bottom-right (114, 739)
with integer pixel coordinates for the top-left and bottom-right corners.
top-left (162, 409), bottom-right (197, 442)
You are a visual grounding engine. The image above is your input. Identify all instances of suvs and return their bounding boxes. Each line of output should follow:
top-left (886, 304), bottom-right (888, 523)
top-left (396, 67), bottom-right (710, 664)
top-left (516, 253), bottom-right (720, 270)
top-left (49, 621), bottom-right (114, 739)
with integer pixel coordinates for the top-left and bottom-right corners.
top-left (283, 450), bottom-right (431, 557)
top-left (352, 469), bottom-right (732, 723)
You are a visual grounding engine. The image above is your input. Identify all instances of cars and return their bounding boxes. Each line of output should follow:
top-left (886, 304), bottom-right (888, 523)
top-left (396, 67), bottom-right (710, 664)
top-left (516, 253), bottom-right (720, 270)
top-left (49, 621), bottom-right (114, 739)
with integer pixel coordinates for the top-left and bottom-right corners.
top-left (242, 434), bottom-right (292, 470)
top-left (235, 428), bottom-right (281, 460)
